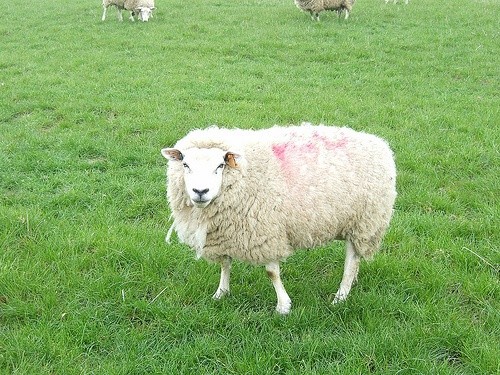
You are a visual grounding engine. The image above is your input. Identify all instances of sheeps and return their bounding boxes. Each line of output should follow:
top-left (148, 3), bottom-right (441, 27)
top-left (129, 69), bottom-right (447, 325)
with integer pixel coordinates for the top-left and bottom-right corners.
top-left (101, 0), bottom-right (157, 24)
top-left (295, 0), bottom-right (355, 23)
top-left (160, 124), bottom-right (398, 315)
